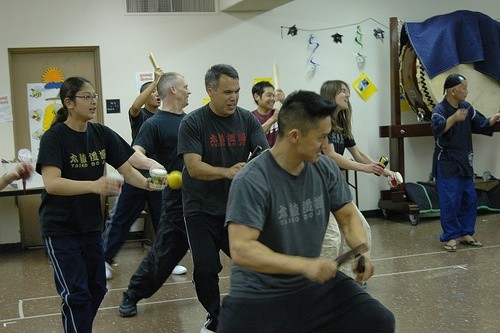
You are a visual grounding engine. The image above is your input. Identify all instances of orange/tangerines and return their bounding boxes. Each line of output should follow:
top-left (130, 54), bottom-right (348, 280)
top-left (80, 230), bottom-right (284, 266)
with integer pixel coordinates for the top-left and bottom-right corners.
top-left (168, 170), bottom-right (182, 189)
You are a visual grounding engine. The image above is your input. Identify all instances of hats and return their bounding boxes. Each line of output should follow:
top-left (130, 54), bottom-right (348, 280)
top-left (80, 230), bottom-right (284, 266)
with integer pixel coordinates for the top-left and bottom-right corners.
top-left (443, 74), bottom-right (466, 95)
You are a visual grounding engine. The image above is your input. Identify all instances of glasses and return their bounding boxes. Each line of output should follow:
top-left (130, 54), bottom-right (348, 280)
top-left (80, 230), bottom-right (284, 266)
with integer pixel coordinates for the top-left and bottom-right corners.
top-left (66, 93), bottom-right (99, 102)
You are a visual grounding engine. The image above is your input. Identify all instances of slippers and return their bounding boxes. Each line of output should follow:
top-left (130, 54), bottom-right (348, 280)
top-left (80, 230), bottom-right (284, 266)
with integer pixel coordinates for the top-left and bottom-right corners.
top-left (444, 244), bottom-right (457, 252)
top-left (458, 238), bottom-right (483, 247)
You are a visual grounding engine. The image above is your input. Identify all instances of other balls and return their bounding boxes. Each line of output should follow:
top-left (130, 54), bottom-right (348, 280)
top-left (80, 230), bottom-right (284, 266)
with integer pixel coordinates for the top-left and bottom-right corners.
top-left (167, 171), bottom-right (182, 189)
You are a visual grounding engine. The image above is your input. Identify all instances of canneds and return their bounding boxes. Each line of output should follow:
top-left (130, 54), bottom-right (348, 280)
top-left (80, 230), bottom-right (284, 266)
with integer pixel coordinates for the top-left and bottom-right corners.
top-left (373, 155), bottom-right (389, 176)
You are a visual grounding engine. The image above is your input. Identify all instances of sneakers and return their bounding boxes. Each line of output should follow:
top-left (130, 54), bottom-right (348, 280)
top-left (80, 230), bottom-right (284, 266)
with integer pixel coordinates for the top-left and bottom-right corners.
top-left (119, 292), bottom-right (143, 317)
top-left (172, 264), bottom-right (187, 275)
top-left (200, 319), bottom-right (218, 333)
top-left (105, 261), bottom-right (116, 279)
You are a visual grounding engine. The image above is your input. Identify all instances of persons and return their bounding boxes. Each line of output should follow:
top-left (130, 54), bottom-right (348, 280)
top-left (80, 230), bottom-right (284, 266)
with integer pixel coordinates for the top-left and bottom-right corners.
top-left (250, 81), bottom-right (286, 148)
top-left (0, 161), bottom-right (32, 192)
top-left (430, 74), bottom-right (500, 252)
top-left (119, 72), bottom-right (223, 317)
top-left (224, 90), bottom-right (372, 333)
top-left (101, 67), bottom-right (189, 280)
top-left (177, 64), bottom-right (270, 333)
top-left (318, 80), bottom-right (403, 289)
top-left (36, 76), bottom-right (167, 333)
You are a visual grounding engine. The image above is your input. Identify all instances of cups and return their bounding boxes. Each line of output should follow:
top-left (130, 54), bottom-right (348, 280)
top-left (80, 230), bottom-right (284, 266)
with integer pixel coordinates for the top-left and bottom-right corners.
top-left (387, 171), bottom-right (405, 189)
top-left (148, 169), bottom-right (168, 191)
top-left (106, 173), bottom-right (124, 195)
